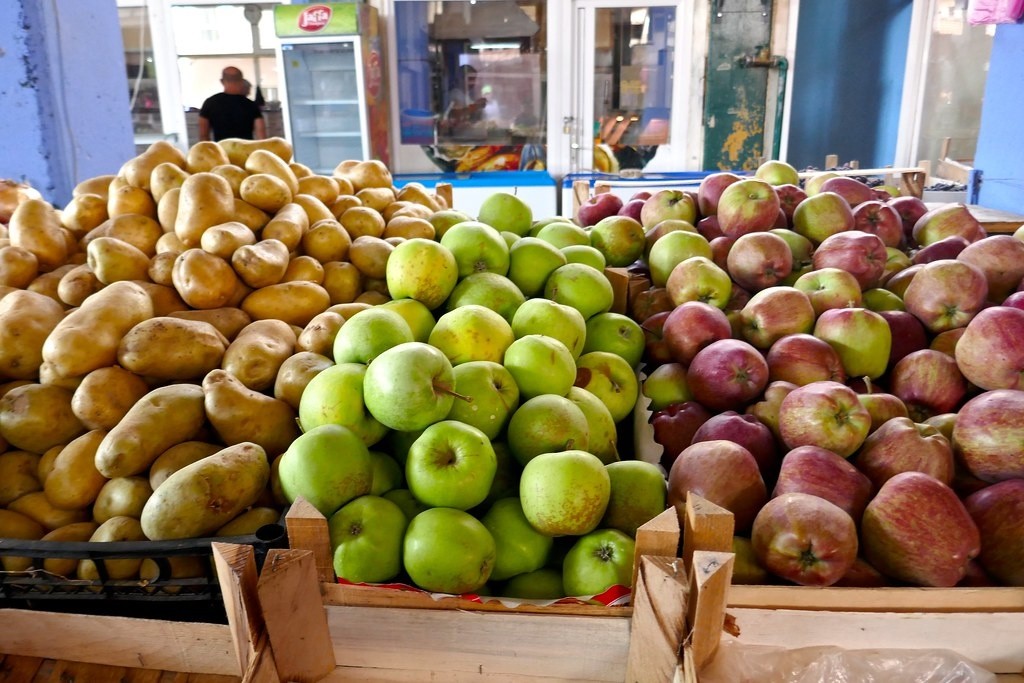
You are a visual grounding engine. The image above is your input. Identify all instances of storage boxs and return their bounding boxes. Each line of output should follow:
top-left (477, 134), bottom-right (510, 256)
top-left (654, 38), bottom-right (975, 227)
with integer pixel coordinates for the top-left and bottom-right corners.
top-left (0, 180), bottom-right (1024, 683)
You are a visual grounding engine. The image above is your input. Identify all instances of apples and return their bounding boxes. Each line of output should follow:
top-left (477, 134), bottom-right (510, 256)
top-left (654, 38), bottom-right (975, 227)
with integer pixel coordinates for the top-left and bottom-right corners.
top-left (278, 160), bottom-right (1024, 599)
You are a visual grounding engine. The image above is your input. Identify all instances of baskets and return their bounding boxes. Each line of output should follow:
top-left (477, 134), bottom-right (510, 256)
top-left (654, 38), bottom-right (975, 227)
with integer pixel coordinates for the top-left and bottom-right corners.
top-left (0, 502), bottom-right (290, 625)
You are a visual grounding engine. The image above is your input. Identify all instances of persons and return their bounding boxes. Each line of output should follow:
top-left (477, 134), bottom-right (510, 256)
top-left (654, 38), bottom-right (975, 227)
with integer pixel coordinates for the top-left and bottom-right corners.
top-left (199, 67), bottom-right (266, 142)
top-left (442, 65), bottom-right (487, 136)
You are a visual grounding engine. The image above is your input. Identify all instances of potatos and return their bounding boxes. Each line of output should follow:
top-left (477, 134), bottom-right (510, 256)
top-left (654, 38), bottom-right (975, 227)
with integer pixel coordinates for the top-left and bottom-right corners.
top-left (0, 137), bottom-right (449, 592)
